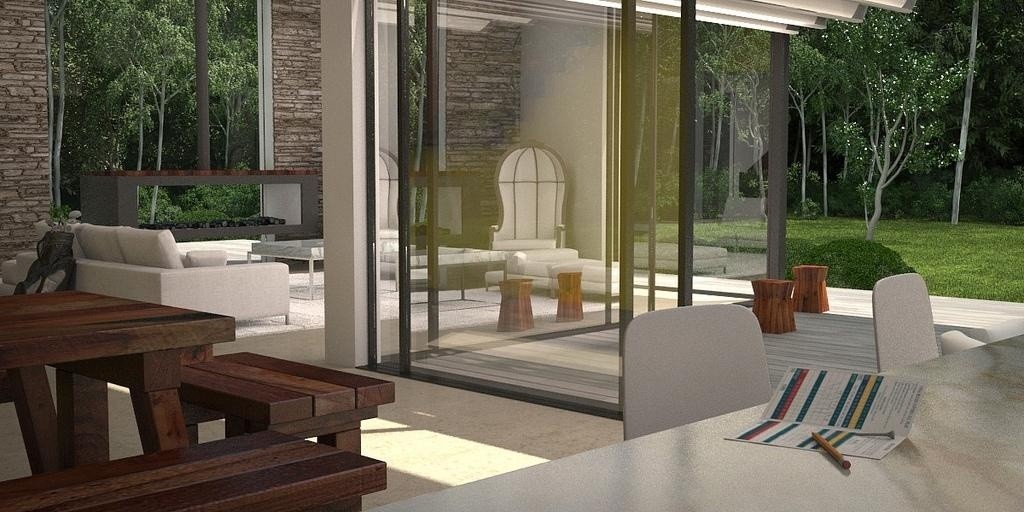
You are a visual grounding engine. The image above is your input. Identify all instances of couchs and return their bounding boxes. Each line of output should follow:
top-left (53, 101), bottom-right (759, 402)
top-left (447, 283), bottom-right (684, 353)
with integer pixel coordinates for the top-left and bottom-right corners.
top-left (485, 249), bottom-right (620, 297)
top-left (1, 220), bottom-right (290, 324)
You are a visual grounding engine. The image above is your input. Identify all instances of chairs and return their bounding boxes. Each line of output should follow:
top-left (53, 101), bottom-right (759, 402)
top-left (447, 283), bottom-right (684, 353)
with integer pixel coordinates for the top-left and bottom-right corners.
top-left (872, 272), bottom-right (935, 372)
top-left (490, 139), bottom-right (570, 248)
top-left (622, 303), bottom-right (770, 440)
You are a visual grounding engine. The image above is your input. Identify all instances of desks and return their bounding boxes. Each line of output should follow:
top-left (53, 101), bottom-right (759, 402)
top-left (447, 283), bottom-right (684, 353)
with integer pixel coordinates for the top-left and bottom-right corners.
top-left (1, 288), bottom-right (236, 474)
top-left (351, 331), bottom-right (1024, 512)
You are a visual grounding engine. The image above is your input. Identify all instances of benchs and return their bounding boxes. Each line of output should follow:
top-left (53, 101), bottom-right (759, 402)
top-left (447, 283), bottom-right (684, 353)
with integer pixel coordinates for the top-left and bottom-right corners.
top-left (633, 243), bottom-right (727, 274)
top-left (0, 430), bottom-right (386, 512)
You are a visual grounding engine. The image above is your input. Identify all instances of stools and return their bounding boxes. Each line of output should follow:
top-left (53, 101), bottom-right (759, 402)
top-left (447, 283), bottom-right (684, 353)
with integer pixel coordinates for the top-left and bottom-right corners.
top-left (499, 271), bottom-right (586, 332)
top-left (182, 351), bottom-right (395, 453)
top-left (751, 264), bottom-right (829, 334)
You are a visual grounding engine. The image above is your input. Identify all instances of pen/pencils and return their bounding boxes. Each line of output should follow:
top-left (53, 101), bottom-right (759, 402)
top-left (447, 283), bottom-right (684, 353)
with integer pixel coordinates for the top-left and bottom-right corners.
top-left (811, 432), bottom-right (851, 469)
